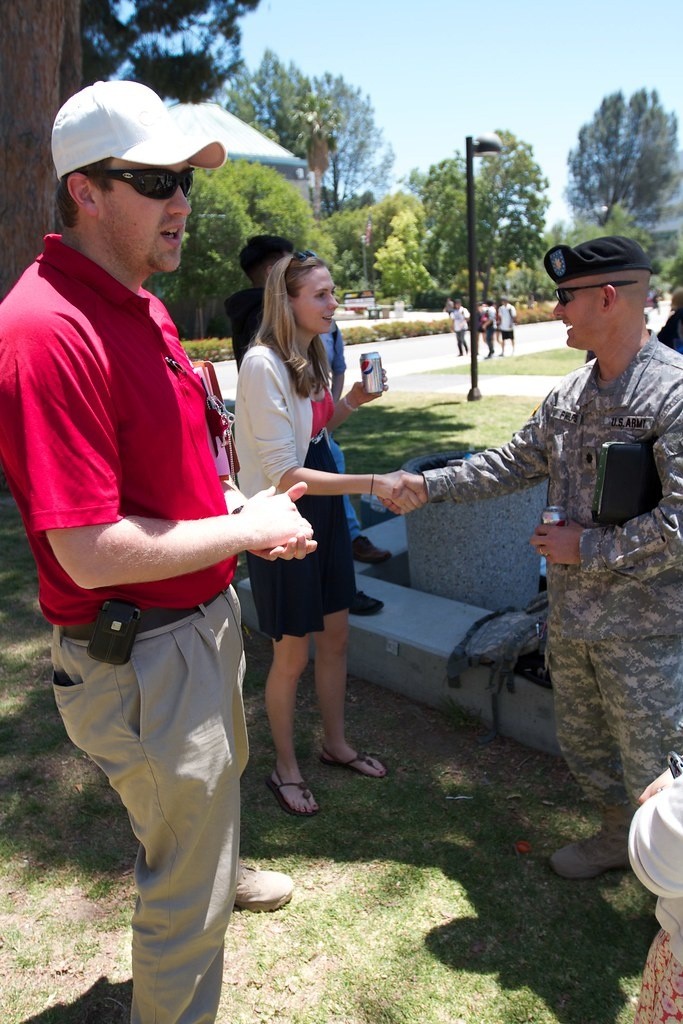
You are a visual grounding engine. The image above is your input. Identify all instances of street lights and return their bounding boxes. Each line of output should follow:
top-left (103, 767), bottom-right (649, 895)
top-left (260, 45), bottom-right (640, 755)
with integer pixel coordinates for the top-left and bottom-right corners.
top-left (464, 135), bottom-right (504, 400)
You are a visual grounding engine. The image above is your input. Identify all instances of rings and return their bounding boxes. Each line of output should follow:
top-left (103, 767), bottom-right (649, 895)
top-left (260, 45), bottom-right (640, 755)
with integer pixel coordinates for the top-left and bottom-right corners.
top-left (540, 548), bottom-right (545, 555)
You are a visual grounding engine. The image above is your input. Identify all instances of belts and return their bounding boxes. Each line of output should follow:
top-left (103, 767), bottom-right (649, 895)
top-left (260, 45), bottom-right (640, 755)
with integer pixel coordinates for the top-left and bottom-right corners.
top-left (58, 591), bottom-right (221, 640)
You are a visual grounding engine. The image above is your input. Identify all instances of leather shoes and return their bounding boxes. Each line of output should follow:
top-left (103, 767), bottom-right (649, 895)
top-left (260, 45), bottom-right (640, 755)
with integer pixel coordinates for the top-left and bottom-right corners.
top-left (353, 536), bottom-right (391, 564)
top-left (351, 593), bottom-right (385, 615)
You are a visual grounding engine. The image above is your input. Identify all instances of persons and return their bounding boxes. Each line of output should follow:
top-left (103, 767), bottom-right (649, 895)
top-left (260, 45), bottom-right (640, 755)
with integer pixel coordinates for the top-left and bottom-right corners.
top-left (0, 80), bottom-right (318, 1024)
top-left (627, 768), bottom-right (683, 1024)
top-left (224, 235), bottom-right (384, 615)
top-left (317, 319), bottom-right (392, 563)
top-left (377, 236), bottom-right (683, 879)
top-left (647, 287), bottom-right (671, 309)
top-left (443, 297), bottom-right (454, 315)
top-left (656, 287), bottom-right (683, 353)
top-left (233, 251), bottom-right (422, 816)
top-left (477, 295), bottom-right (517, 359)
top-left (449, 299), bottom-right (470, 357)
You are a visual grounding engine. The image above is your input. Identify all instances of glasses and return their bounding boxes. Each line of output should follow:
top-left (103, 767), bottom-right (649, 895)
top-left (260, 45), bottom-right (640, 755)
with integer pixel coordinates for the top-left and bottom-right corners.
top-left (104, 168), bottom-right (194, 200)
top-left (292, 250), bottom-right (317, 262)
top-left (556, 279), bottom-right (638, 306)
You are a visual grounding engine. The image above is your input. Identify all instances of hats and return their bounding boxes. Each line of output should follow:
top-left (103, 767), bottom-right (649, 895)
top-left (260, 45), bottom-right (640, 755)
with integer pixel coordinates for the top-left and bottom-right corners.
top-left (52, 81), bottom-right (224, 181)
top-left (544, 237), bottom-right (653, 285)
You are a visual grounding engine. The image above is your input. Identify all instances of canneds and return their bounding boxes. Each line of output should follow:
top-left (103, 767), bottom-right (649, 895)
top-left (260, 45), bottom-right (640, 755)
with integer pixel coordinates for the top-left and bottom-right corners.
top-left (541, 506), bottom-right (568, 526)
top-left (360, 351), bottom-right (384, 393)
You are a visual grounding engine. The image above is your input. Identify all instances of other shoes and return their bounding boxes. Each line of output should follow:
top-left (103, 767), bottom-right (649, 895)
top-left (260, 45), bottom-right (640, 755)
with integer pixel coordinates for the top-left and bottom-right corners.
top-left (549, 826), bottom-right (629, 879)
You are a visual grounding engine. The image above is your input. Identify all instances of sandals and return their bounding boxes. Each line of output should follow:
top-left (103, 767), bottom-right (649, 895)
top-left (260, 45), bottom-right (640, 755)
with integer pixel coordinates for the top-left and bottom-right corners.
top-left (319, 745), bottom-right (389, 778)
top-left (265, 764), bottom-right (320, 817)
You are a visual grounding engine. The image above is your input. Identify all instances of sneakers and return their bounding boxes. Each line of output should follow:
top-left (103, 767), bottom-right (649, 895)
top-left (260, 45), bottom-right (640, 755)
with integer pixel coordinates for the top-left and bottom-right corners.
top-left (233, 865), bottom-right (294, 913)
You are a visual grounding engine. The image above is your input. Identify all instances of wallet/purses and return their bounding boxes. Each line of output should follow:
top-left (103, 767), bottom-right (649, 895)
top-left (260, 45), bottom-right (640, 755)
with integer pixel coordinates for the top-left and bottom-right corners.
top-left (592, 439), bottom-right (659, 525)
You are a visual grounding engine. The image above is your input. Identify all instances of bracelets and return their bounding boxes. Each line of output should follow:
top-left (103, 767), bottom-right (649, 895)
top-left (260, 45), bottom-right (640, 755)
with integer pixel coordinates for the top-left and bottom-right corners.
top-left (370, 474), bottom-right (374, 495)
